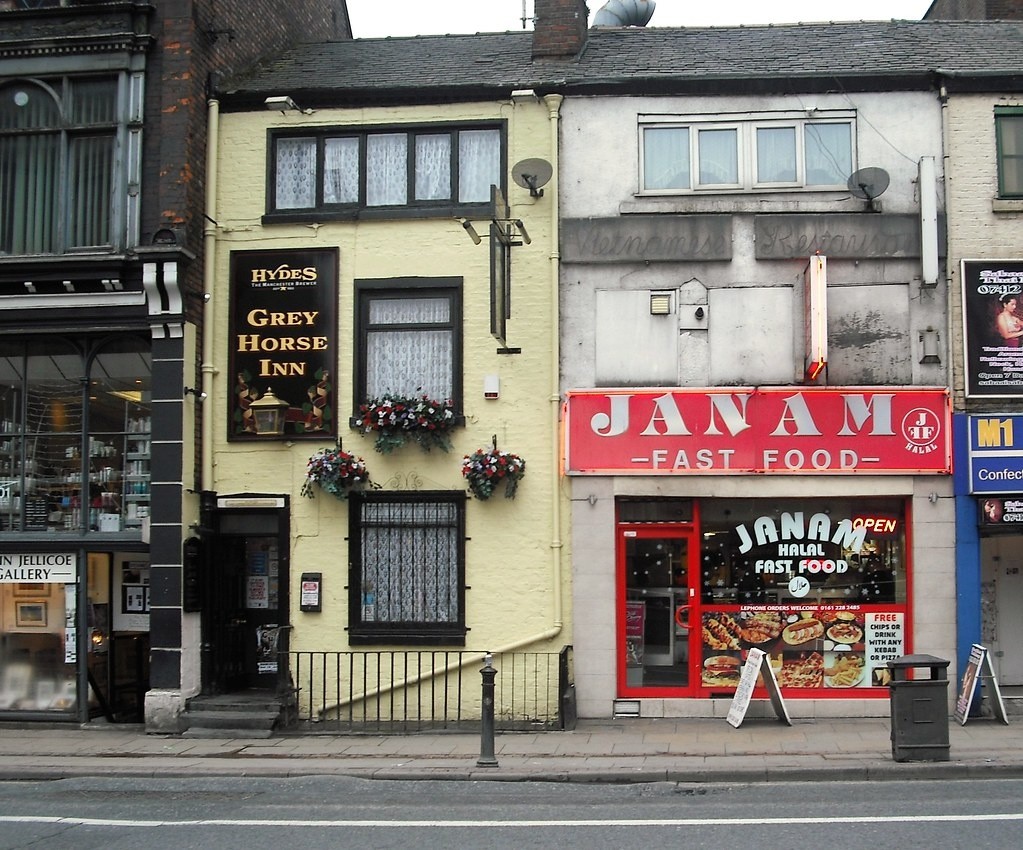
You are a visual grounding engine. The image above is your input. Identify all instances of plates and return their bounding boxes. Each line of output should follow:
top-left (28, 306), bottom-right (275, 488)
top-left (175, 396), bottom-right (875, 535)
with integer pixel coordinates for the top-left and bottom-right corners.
top-left (825, 670), bottom-right (865, 689)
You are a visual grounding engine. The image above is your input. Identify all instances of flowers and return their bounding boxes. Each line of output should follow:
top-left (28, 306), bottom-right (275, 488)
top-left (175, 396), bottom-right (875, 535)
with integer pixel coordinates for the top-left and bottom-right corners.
top-left (462, 449), bottom-right (525, 503)
top-left (355, 386), bottom-right (458, 455)
top-left (300, 438), bottom-right (383, 500)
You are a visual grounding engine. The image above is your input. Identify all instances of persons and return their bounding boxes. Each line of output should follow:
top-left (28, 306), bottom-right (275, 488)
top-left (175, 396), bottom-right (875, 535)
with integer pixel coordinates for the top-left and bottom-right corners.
top-left (663, 568), bottom-right (689, 648)
top-left (699, 562), bottom-right (715, 604)
top-left (737, 567), bottom-right (775, 604)
top-left (996, 293), bottom-right (1023, 348)
top-left (825, 553), bottom-right (896, 603)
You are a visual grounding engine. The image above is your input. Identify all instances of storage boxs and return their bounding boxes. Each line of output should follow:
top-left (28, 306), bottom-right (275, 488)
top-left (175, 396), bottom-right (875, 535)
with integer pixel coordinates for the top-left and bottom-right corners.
top-left (97, 513), bottom-right (120, 533)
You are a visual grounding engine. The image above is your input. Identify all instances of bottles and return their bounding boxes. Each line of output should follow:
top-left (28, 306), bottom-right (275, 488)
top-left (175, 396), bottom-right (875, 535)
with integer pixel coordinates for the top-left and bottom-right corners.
top-left (66, 466), bottom-right (115, 483)
top-left (125, 481), bottom-right (147, 494)
top-left (3, 437), bottom-right (21, 451)
top-left (127, 501), bottom-right (151, 519)
top-left (127, 440), bottom-right (150, 452)
top-left (64, 506), bottom-right (105, 528)
top-left (130, 461), bottom-right (150, 475)
top-left (0, 455), bottom-right (32, 470)
top-left (0, 473), bottom-right (37, 491)
top-left (65, 434), bottom-right (117, 459)
top-left (1, 417), bottom-right (31, 433)
top-left (128, 415), bottom-right (151, 432)
top-left (101, 490), bottom-right (122, 506)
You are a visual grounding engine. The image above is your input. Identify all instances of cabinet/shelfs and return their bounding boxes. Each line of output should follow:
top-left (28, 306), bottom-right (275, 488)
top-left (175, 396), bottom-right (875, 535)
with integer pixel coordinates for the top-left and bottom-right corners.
top-left (51, 456), bottom-right (122, 513)
top-left (0, 431), bottom-right (37, 515)
top-left (121, 434), bottom-right (152, 525)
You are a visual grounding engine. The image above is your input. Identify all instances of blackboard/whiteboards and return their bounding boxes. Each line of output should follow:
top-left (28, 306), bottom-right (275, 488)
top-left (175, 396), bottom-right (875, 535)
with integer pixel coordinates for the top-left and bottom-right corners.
top-left (953, 643), bottom-right (987, 727)
top-left (726, 647), bottom-right (766, 729)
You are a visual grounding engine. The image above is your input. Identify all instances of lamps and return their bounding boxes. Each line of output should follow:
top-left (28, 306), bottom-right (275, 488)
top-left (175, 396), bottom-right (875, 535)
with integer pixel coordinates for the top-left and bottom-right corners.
top-left (509, 88), bottom-right (541, 108)
top-left (92, 627), bottom-right (108, 645)
top-left (264, 97), bottom-right (308, 114)
top-left (247, 386), bottom-right (290, 435)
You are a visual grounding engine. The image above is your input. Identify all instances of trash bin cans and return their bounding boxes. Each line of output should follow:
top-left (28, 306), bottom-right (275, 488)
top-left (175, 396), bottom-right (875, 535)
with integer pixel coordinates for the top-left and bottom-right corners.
top-left (887, 654), bottom-right (950, 762)
